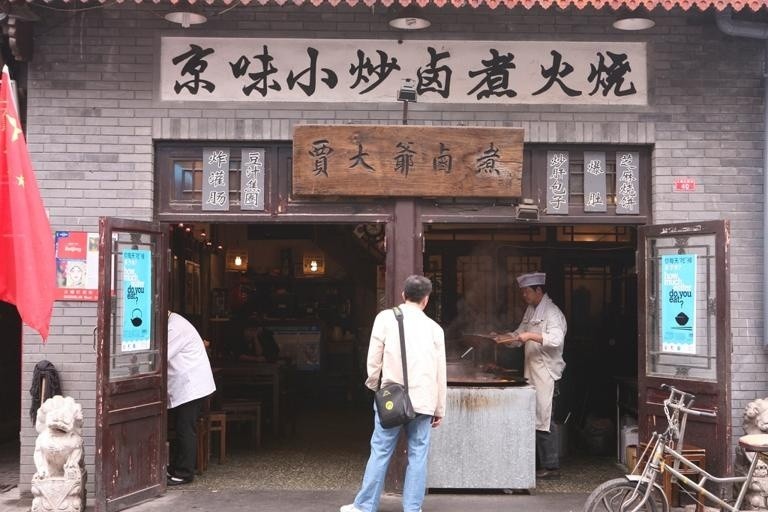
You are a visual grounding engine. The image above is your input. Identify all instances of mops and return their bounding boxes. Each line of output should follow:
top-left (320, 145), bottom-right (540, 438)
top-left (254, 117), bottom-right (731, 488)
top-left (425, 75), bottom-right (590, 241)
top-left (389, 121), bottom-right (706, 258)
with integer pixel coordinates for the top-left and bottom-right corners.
top-left (28, 360), bottom-right (63, 428)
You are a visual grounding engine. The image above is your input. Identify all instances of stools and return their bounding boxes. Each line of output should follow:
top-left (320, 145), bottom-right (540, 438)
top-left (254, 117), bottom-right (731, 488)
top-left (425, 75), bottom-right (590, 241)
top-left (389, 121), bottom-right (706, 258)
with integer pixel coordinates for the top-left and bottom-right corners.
top-left (191, 397), bottom-right (263, 473)
top-left (640, 440), bottom-right (708, 511)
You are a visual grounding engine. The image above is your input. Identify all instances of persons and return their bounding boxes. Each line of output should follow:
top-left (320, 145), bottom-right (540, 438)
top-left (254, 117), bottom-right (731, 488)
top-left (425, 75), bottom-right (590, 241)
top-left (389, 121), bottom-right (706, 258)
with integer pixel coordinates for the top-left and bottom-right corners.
top-left (225, 318), bottom-right (280, 419)
top-left (331, 296), bottom-right (357, 405)
top-left (339, 276), bottom-right (448, 512)
top-left (167, 309), bottom-right (217, 485)
top-left (489, 273), bottom-right (569, 479)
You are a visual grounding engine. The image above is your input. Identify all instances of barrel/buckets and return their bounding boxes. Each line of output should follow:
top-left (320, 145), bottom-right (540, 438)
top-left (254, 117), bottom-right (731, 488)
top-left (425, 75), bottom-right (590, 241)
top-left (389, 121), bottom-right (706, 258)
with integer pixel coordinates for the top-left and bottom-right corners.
top-left (620, 424), bottom-right (639, 464)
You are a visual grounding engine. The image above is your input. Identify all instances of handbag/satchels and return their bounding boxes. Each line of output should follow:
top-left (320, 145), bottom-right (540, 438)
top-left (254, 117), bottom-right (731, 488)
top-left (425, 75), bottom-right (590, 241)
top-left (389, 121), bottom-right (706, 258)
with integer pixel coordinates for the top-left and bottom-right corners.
top-left (374, 383), bottom-right (416, 429)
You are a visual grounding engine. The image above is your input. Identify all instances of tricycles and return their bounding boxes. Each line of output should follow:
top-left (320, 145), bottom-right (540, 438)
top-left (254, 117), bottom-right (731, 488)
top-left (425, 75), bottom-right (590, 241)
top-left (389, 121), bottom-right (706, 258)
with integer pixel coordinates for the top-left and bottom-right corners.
top-left (581, 382), bottom-right (768, 512)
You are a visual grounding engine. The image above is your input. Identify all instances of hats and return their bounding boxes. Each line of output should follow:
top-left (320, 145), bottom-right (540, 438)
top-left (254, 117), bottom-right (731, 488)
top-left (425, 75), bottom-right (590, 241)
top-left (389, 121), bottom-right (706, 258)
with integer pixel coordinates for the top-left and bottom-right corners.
top-left (198, 331), bottom-right (217, 341)
top-left (515, 272), bottom-right (547, 289)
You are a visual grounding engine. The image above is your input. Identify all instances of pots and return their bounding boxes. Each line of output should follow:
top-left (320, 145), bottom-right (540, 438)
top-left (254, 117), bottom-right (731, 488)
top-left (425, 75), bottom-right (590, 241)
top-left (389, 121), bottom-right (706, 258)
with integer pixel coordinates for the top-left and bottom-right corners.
top-left (462, 334), bottom-right (519, 352)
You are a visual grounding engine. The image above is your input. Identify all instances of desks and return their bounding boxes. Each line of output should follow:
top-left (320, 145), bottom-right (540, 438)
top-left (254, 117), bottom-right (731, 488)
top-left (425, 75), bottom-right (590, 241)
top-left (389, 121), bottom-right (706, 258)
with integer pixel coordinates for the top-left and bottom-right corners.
top-left (209, 361), bottom-right (282, 440)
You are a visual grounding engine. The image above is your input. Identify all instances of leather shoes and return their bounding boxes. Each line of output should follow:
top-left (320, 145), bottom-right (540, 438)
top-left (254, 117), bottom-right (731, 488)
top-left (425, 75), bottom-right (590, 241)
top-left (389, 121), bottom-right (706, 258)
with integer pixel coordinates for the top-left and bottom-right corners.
top-left (536, 467), bottom-right (561, 480)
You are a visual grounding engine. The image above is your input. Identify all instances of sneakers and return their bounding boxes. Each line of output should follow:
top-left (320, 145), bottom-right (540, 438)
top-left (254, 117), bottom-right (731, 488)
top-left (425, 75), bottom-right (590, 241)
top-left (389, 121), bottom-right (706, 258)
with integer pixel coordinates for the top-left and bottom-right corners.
top-left (340, 503), bottom-right (363, 512)
top-left (166, 471), bottom-right (193, 486)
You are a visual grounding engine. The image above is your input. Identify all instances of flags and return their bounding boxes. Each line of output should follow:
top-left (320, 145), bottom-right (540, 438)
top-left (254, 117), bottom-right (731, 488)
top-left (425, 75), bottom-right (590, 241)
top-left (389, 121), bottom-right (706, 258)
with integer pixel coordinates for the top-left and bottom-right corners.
top-left (0, 72), bottom-right (55, 344)
